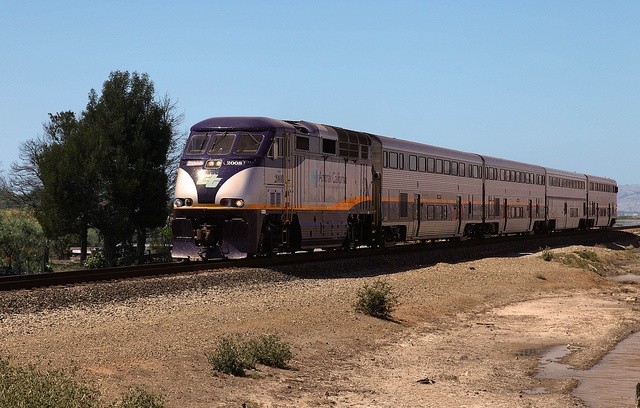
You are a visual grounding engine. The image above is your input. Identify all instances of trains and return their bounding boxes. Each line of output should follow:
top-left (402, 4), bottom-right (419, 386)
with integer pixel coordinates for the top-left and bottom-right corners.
top-left (171, 117), bottom-right (618, 264)
